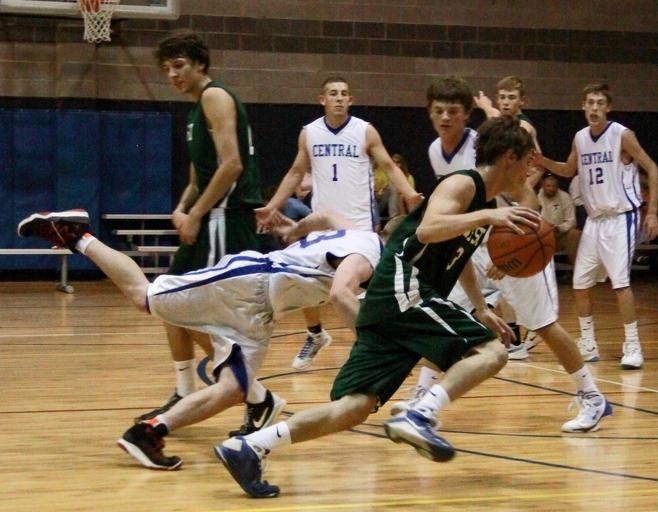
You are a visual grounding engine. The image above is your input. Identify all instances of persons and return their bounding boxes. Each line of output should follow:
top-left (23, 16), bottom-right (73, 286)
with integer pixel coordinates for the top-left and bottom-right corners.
top-left (473, 76), bottom-right (547, 360)
top-left (252, 77), bottom-right (426, 372)
top-left (134, 32), bottom-right (284, 437)
top-left (390, 80), bottom-right (613, 433)
top-left (524, 83), bottom-right (657, 371)
top-left (259, 154), bottom-right (657, 289)
top-left (213, 112), bottom-right (543, 498)
top-left (17, 208), bottom-right (410, 470)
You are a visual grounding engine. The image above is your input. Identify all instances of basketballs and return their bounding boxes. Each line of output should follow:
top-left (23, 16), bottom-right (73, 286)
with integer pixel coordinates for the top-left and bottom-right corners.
top-left (487, 217), bottom-right (555, 277)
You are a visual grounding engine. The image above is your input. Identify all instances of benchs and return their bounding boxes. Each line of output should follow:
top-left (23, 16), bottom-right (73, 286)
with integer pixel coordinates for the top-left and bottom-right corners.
top-left (553, 244), bottom-right (658, 270)
top-left (1, 249), bottom-right (74, 293)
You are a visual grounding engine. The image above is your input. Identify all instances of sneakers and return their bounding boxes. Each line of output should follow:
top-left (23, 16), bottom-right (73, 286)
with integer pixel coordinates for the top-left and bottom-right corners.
top-left (501, 329), bottom-right (644, 433)
top-left (16, 208), bottom-right (91, 254)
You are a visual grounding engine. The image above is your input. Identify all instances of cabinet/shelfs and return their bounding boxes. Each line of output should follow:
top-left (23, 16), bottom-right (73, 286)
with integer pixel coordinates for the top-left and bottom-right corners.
top-left (101, 212), bottom-right (181, 255)
